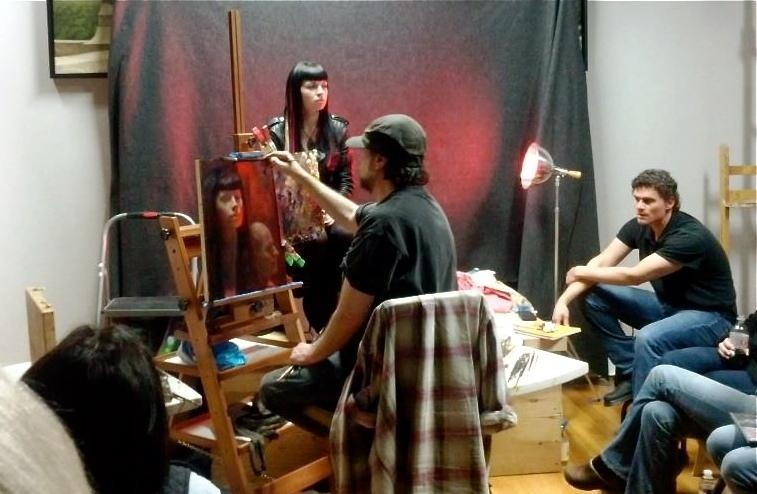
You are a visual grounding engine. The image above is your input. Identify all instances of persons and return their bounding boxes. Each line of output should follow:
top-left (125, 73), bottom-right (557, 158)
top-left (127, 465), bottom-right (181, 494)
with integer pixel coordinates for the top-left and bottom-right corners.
top-left (22, 323), bottom-right (224, 494)
top-left (562, 364), bottom-right (757, 493)
top-left (258, 112), bottom-right (457, 443)
top-left (550, 165), bottom-right (734, 406)
top-left (658, 309), bottom-right (757, 397)
top-left (202, 164), bottom-right (292, 320)
top-left (267, 57), bottom-right (356, 344)
top-left (704, 423), bottom-right (757, 493)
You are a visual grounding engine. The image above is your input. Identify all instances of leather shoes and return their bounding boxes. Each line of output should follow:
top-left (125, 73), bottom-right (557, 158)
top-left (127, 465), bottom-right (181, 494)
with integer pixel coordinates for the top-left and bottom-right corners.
top-left (604, 381), bottom-right (631, 402)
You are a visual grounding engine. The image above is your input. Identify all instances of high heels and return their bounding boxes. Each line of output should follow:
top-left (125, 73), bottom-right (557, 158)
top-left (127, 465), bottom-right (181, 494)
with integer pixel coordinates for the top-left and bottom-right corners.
top-left (564, 459), bottom-right (609, 490)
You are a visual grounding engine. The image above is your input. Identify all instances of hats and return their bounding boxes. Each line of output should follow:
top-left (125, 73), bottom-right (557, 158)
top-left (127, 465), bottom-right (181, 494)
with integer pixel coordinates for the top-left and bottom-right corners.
top-left (345, 113), bottom-right (426, 156)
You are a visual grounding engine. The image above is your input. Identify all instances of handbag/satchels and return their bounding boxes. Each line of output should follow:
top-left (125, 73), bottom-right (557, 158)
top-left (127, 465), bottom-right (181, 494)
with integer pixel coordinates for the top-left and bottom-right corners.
top-left (272, 115), bottom-right (327, 247)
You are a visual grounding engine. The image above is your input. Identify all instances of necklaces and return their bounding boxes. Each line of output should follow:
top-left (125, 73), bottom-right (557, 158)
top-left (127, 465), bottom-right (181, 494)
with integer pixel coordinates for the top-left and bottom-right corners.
top-left (301, 124), bottom-right (319, 150)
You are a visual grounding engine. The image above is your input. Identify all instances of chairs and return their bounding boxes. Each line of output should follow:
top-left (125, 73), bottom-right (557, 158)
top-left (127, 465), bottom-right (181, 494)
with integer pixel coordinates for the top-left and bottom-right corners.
top-left (25, 286), bottom-right (57, 368)
top-left (304, 290), bottom-right (484, 493)
top-left (95, 211), bottom-right (198, 330)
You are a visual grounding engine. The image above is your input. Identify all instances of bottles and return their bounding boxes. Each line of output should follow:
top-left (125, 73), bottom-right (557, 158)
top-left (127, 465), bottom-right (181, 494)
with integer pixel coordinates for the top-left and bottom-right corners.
top-left (698, 470), bottom-right (720, 494)
top-left (561, 420), bottom-right (570, 467)
top-left (726, 314), bottom-right (749, 368)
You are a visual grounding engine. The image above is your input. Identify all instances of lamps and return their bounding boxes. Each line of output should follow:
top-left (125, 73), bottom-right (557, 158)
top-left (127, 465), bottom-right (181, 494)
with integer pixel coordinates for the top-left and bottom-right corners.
top-left (521, 142), bottom-right (601, 402)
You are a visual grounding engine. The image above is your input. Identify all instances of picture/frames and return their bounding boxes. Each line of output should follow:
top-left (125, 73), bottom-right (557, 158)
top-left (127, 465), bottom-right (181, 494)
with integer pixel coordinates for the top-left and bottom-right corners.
top-left (46, 0), bottom-right (113, 78)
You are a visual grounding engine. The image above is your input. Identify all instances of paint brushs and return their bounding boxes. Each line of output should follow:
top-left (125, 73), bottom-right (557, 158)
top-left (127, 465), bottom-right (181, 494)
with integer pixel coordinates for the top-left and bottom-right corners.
top-left (276, 327), bottom-right (324, 384)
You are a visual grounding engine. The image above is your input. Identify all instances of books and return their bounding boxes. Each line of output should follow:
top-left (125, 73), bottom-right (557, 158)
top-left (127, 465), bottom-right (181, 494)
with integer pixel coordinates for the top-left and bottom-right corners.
top-left (513, 320), bottom-right (583, 342)
top-left (728, 409), bottom-right (757, 444)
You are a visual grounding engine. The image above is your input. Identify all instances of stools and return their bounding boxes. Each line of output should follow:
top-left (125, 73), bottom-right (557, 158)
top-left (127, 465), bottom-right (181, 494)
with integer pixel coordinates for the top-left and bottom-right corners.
top-left (681, 437), bottom-right (721, 477)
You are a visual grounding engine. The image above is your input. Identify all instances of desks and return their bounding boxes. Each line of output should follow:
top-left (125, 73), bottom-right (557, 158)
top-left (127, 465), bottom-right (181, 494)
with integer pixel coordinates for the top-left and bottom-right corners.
top-left (488, 317), bottom-right (567, 477)
